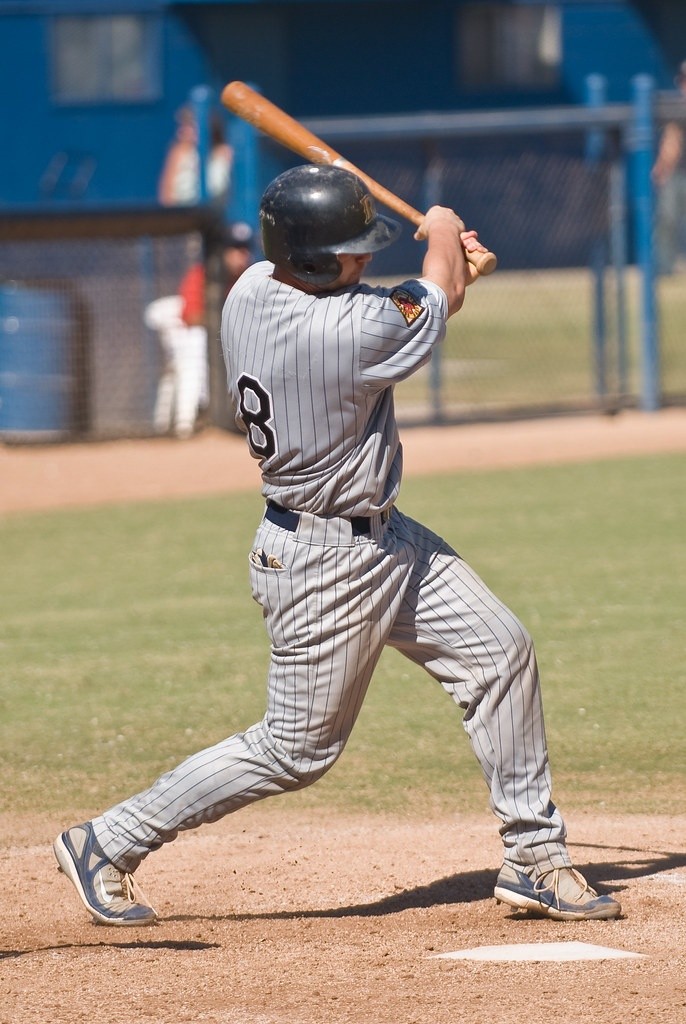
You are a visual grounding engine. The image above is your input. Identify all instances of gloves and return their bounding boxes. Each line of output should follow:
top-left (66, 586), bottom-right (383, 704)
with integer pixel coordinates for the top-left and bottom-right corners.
top-left (250, 548), bottom-right (287, 569)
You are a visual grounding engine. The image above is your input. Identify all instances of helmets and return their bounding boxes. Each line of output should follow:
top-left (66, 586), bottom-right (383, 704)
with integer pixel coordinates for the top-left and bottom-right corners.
top-left (260, 164), bottom-right (402, 285)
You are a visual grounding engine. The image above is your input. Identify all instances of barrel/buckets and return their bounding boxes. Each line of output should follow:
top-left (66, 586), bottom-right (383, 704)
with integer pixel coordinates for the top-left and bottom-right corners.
top-left (4, 279), bottom-right (88, 444)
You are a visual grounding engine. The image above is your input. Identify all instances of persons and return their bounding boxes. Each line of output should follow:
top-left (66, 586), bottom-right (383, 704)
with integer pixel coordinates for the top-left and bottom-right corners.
top-left (53, 156), bottom-right (620, 928)
top-left (145, 222), bottom-right (256, 435)
top-left (650, 63), bottom-right (686, 179)
top-left (161, 106), bottom-right (235, 199)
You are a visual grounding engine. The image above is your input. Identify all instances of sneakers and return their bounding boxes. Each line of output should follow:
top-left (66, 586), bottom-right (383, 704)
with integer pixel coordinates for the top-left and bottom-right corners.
top-left (494, 862), bottom-right (621, 921)
top-left (53, 820), bottom-right (159, 925)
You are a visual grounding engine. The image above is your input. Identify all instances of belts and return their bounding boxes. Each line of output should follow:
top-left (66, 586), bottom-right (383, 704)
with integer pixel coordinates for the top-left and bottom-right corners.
top-left (265, 501), bottom-right (389, 536)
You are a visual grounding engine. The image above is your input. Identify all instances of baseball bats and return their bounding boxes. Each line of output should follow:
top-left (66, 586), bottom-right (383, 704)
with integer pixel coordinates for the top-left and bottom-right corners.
top-left (219, 79), bottom-right (497, 276)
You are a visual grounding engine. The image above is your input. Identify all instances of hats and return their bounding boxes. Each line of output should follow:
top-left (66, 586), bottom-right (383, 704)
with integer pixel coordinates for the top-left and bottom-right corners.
top-left (227, 223), bottom-right (255, 248)
